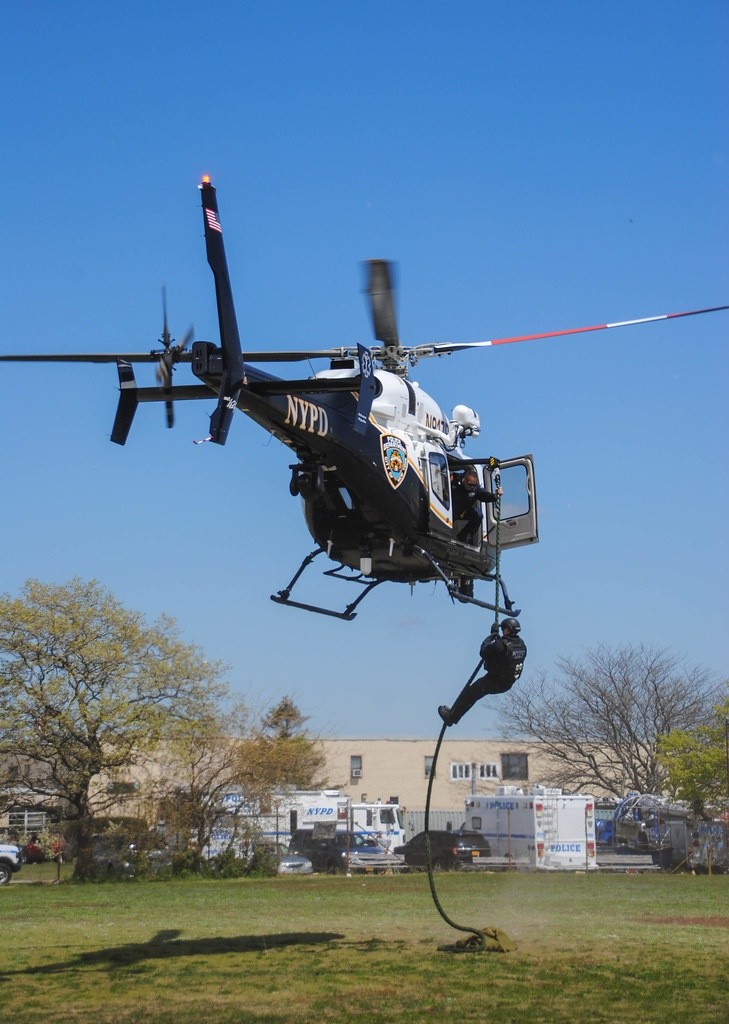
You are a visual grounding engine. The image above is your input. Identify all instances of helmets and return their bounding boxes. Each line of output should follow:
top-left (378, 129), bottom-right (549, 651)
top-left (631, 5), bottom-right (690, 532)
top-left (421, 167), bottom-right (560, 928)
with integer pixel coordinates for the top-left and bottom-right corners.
top-left (501, 618), bottom-right (522, 632)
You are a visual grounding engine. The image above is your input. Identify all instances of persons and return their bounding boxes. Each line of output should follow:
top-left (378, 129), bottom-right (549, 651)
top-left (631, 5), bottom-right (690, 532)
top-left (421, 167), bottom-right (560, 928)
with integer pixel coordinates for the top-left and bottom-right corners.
top-left (451, 468), bottom-right (503, 544)
top-left (438, 618), bottom-right (527, 727)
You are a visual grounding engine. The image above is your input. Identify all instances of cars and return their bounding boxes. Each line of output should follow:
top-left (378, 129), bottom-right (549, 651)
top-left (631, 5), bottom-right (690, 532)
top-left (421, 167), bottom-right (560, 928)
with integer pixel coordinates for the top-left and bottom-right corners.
top-left (251, 839), bottom-right (314, 876)
top-left (0, 842), bottom-right (23, 885)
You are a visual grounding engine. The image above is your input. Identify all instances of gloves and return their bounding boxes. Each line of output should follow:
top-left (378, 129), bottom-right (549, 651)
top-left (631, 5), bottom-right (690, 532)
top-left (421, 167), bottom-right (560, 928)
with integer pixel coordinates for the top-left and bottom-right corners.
top-left (491, 623), bottom-right (500, 633)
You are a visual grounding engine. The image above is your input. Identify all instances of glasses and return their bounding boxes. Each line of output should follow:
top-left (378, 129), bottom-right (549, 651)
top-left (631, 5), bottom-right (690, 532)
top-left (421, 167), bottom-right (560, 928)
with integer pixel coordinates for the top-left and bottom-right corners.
top-left (466, 477), bottom-right (478, 487)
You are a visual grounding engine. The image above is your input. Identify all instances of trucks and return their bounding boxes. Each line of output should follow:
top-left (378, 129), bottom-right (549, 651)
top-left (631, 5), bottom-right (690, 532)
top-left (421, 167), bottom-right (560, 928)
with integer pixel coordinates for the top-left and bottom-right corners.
top-left (595, 793), bottom-right (729, 875)
top-left (290, 830), bottom-right (386, 872)
top-left (456, 784), bottom-right (599, 875)
top-left (196, 790), bottom-right (408, 866)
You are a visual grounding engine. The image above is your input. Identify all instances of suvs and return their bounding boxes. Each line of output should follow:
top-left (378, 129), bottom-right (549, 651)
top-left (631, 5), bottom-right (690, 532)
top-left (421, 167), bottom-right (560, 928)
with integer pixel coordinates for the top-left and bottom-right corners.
top-left (394, 829), bottom-right (491, 873)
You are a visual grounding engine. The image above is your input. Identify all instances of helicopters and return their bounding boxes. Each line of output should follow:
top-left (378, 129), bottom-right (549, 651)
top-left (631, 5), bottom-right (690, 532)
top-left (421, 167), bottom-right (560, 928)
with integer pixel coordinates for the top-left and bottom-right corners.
top-left (1, 176), bottom-right (729, 620)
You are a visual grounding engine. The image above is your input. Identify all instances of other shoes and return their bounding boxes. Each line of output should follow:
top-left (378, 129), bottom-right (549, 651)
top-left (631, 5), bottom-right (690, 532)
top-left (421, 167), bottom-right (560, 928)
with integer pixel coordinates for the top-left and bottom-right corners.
top-left (438, 705), bottom-right (457, 727)
top-left (457, 530), bottom-right (466, 541)
